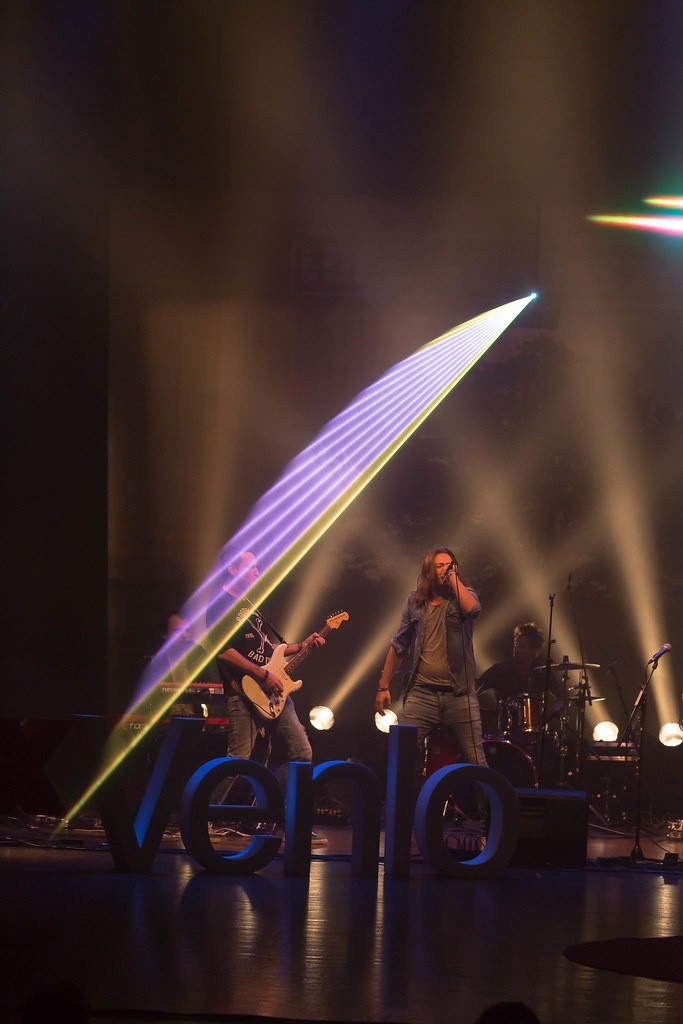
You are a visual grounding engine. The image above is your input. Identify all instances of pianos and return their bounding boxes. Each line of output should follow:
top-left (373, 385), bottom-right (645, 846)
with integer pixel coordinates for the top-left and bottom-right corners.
top-left (103, 678), bottom-right (230, 740)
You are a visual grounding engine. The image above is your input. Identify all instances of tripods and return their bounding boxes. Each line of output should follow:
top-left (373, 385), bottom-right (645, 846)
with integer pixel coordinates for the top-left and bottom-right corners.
top-left (587, 661), bottom-right (683, 873)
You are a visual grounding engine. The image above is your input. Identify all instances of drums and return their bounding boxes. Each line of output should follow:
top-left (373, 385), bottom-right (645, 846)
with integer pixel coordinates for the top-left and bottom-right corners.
top-left (496, 692), bottom-right (545, 736)
top-left (449, 737), bottom-right (539, 831)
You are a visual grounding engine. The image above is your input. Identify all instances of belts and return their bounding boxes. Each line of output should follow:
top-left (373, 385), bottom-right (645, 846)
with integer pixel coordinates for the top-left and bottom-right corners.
top-left (422, 683), bottom-right (454, 692)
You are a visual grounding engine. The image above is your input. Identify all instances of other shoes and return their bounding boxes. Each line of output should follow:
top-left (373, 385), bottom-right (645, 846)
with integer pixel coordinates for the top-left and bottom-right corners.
top-left (411, 835), bottom-right (424, 862)
top-left (277, 827), bottom-right (328, 845)
top-left (479, 815), bottom-right (487, 833)
top-left (207, 821), bottom-right (231, 835)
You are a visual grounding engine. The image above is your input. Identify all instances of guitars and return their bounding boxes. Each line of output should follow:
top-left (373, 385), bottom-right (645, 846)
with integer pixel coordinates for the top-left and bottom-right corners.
top-left (239, 608), bottom-right (351, 725)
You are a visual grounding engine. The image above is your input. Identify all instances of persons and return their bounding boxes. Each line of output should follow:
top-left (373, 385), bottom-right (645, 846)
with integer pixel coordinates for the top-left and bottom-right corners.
top-left (375, 546), bottom-right (490, 834)
top-left (476, 624), bottom-right (571, 790)
top-left (143, 611), bottom-right (216, 837)
top-left (207, 547), bottom-right (328, 844)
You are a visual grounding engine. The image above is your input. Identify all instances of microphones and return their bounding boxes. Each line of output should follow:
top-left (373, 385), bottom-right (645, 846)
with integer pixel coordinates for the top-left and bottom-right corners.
top-left (648, 643), bottom-right (672, 664)
top-left (545, 706), bottom-right (563, 725)
top-left (444, 561), bottom-right (454, 576)
top-left (605, 659), bottom-right (619, 676)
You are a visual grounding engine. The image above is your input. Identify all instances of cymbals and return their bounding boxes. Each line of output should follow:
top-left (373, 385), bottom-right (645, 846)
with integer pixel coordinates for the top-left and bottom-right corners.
top-left (534, 655), bottom-right (601, 673)
top-left (553, 692), bottom-right (608, 704)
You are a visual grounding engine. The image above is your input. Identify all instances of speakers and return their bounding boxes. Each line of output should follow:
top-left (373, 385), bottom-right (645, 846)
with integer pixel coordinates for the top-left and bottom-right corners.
top-left (513, 787), bottom-right (587, 871)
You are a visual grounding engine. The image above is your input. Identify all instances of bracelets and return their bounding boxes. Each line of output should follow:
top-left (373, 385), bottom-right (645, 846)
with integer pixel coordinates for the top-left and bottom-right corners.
top-left (298, 642), bottom-right (301, 652)
top-left (448, 570), bottom-right (456, 576)
top-left (377, 688), bottom-right (390, 691)
top-left (260, 670), bottom-right (269, 682)
